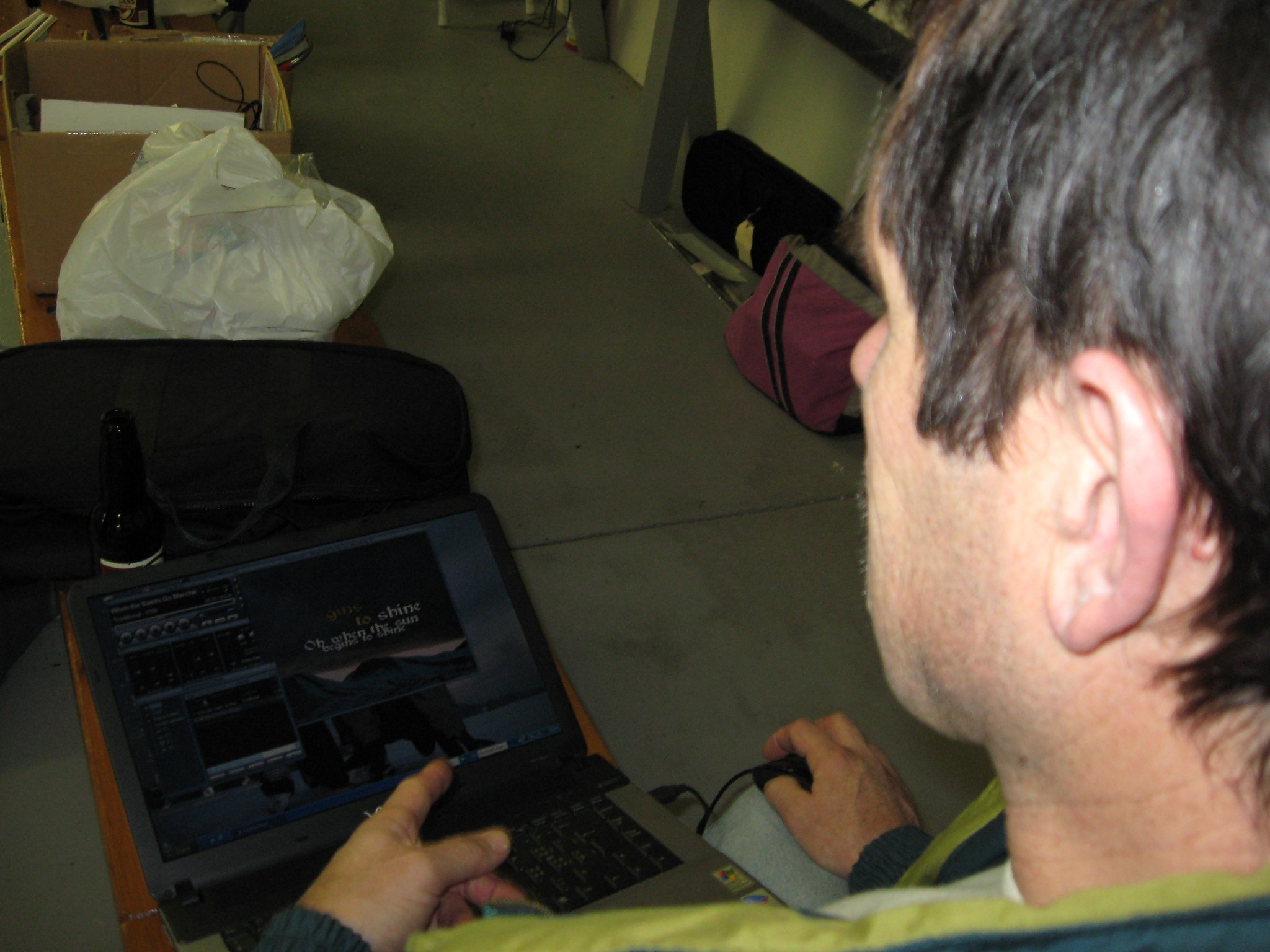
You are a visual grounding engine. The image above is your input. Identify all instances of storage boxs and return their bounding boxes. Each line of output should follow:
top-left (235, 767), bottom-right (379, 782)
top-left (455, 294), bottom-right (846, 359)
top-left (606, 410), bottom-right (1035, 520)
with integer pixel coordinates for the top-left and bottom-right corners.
top-left (4, 33), bottom-right (296, 294)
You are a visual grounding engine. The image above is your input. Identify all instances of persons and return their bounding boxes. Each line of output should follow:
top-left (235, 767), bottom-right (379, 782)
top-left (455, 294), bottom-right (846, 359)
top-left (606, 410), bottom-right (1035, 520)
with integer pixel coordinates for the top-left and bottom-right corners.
top-left (263, 0), bottom-right (1270, 952)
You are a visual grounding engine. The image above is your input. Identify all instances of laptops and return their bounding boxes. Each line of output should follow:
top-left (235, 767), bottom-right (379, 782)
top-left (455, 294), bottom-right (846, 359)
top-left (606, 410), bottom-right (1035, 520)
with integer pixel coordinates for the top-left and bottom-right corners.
top-left (68, 491), bottom-right (790, 952)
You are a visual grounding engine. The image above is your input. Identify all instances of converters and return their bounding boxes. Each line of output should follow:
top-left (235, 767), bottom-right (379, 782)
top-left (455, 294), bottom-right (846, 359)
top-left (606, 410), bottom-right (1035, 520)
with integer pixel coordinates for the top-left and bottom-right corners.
top-left (500, 21), bottom-right (516, 40)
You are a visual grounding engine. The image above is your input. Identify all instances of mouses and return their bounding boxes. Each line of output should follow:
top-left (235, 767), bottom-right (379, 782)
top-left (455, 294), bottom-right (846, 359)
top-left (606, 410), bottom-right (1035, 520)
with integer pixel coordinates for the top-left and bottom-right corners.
top-left (752, 752), bottom-right (815, 796)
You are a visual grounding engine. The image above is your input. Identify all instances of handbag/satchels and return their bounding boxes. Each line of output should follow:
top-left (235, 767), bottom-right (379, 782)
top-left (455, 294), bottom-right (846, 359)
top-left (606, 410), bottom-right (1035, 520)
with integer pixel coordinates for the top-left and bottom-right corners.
top-left (54, 125), bottom-right (394, 343)
top-left (724, 230), bottom-right (894, 438)
